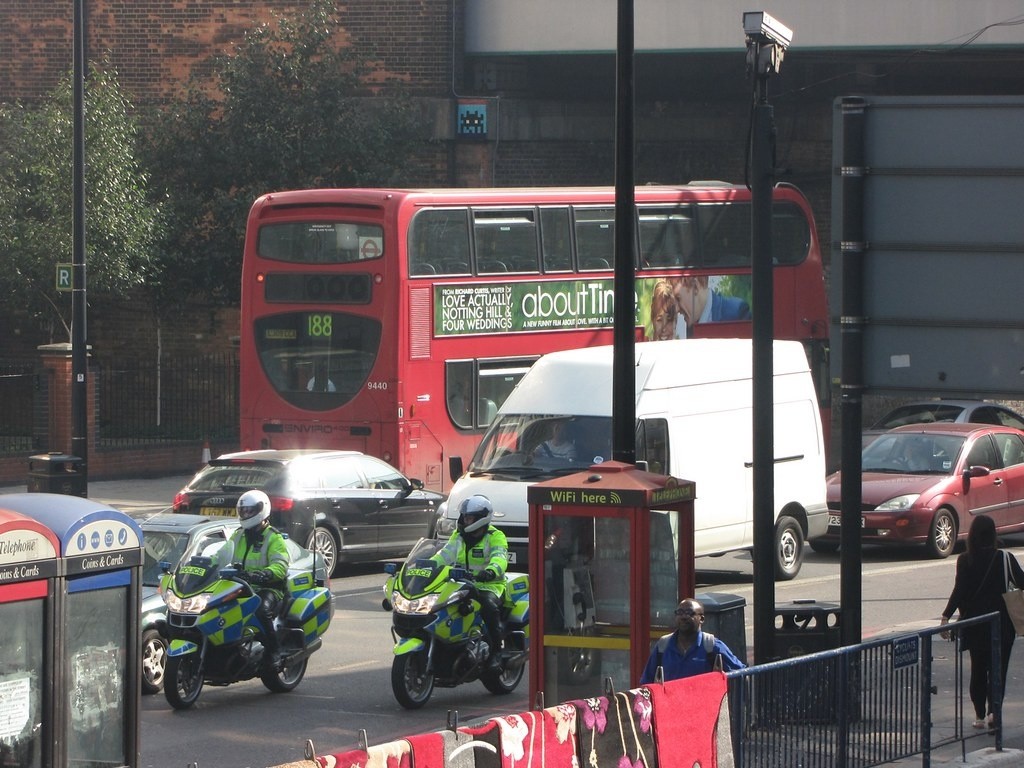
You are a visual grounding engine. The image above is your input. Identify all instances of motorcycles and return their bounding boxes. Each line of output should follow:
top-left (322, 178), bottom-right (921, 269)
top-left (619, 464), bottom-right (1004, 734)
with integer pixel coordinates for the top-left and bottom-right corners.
top-left (158, 538), bottom-right (335, 711)
top-left (382, 536), bottom-right (528, 711)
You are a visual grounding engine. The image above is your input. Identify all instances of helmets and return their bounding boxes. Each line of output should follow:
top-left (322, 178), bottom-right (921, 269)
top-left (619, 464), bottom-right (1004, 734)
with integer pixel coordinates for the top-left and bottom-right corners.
top-left (455, 495), bottom-right (492, 534)
top-left (236, 490), bottom-right (271, 529)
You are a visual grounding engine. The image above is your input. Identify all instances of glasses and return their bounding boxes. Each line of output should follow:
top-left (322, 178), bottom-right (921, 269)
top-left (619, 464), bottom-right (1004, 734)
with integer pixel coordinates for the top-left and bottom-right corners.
top-left (674, 609), bottom-right (702, 616)
top-left (240, 509), bottom-right (256, 514)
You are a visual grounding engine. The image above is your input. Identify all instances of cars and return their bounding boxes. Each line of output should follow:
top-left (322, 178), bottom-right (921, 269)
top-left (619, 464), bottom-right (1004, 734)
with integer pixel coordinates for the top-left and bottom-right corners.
top-left (132, 514), bottom-right (330, 695)
top-left (860, 398), bottom-right (1024, 450)
top-left (171, 448), bottom-right (450, 579)
top-left (809, 423), bottom-right (1024, 560)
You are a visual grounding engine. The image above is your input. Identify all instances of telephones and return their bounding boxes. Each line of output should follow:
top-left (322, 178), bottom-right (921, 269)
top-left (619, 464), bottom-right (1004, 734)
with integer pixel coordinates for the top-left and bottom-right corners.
top-left (563, 567), bottom-right (596, 628)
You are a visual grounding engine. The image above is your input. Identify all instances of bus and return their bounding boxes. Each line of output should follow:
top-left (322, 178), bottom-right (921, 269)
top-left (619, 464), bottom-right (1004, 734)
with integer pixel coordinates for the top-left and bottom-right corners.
top-left (238, 180), bottom-right (834, 496)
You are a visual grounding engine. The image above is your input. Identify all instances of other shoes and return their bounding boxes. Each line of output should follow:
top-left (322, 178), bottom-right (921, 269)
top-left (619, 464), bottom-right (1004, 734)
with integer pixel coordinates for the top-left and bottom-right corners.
top-left (486, 647), bottom-right (502, 669)
top-left (267, 654), bottom-right (282, 673)
top-left (988, 713), bottom-right (994, 734)
top-left (971, 720), bottom-right (984, 727)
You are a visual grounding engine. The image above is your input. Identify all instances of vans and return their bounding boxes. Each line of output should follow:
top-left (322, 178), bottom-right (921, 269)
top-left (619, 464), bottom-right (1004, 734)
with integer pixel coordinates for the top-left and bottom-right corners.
top-left (435, 338), bottom-right (829, 582)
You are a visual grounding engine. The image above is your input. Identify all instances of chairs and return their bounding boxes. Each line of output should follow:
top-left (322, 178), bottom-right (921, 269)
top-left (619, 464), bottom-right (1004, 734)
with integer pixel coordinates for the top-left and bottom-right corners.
top-left (437, 257), bottom-right (469, 274)
top-left (515, 258), bottom-right (537, 271)
top-left (583, 256), bottom-right (610, 269)
top-left (642, 256), bottom-right (652, 267)
top-left (548, 257), bottom-right (570, 270)
top-left (478, 260), bottom-right (508, 273)
top-left (411, 261), bottom-right (438, 276)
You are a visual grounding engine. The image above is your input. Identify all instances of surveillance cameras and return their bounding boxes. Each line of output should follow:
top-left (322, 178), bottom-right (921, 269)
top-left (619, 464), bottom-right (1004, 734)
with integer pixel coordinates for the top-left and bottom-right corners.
top-left (741, 11), bottom-right (794, 50)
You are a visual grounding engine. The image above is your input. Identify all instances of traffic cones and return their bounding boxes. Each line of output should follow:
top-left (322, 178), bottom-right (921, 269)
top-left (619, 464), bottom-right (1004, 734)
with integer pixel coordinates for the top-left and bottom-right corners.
top-left (201, 434), bottom-right (212, 468)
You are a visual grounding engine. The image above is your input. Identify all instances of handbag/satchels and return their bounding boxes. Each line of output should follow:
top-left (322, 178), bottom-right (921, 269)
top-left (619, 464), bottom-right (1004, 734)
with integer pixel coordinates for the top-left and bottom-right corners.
top-left (1002, 551), bottom-right (1024, 636)
top-left (950, 616), bottom-right (964, 641)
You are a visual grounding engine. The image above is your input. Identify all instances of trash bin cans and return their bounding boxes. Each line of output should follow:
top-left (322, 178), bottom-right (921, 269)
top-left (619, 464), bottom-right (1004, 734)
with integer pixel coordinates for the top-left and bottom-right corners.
top-left (696, 592), bottom-right (746, 669)
top-left (771, 600), bottom-right (842, 725)
top-left (27, 453), bottom-right (83, 496)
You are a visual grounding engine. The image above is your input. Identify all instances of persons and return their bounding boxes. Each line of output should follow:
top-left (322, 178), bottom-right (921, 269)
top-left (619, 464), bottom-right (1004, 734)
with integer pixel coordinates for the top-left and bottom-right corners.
top-left (307, 358), bottom-right (336, 392)
top-left (651, 275), bottom-right (751, 341)
top-left (640, 598), bottom-right (748, 690)
top-left (528, 420), bottom-right (577, 459)
top-left (939, 516), bottom-right (1024, 734)
top-left (405, 495), bottom-right (508, 670)
top-left (893, 441), bottom-right (930, 470)
top-left (211, 490), bottom-right (290, 674)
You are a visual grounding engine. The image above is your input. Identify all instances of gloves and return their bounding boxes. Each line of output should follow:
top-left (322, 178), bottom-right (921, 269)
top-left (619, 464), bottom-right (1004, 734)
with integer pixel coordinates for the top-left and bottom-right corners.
top-left (250, 570), bottom-right (268, 584)
top-left (477, 570), bottom-right (495, 582)
